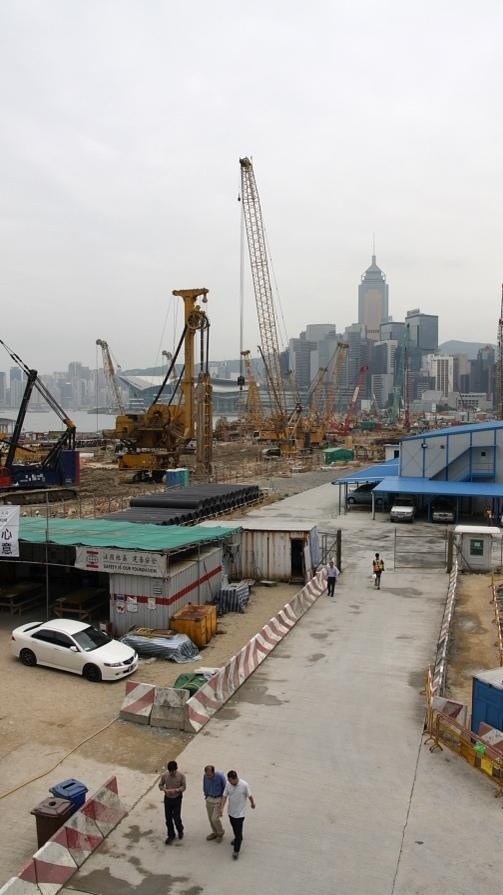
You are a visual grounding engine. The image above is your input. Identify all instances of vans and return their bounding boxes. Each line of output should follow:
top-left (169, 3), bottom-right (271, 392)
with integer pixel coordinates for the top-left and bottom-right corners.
top-left (431, 500), bottom-right (455, 524)
top-left (343, 483), bottom-right (389, 505)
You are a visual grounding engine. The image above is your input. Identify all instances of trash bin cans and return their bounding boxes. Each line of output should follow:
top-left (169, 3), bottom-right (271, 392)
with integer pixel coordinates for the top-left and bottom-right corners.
top-left (30, 797), bottom-right (76, 850)
top-left (48, 778), bottom-right (89, 813)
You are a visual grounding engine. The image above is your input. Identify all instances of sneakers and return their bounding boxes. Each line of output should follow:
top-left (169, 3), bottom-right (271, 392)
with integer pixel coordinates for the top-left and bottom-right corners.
top-left (374, 584), bottom-right (380, 590)
top-left (206, 830), bottom-right (225, 843)
top-left (231, 838), bottom-right (239, 859)
top-left (165, 831), bottom-right (184, 845)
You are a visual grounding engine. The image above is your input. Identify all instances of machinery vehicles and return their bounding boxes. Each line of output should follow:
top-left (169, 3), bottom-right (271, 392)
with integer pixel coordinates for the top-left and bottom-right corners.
top-left (0, 338), bottom-right (80, 504)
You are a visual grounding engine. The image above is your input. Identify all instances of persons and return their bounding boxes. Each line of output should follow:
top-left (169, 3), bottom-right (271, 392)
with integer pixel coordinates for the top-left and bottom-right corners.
top-left (373, 553), bottom-right (384, 590)
top-left (203, 765), bottom-right (227, 843)
top-left (220, 770), bottom-right (256, 860)
top-left (484, 505), bottom-right (493, 526)
top-left (327, 562), bottom-right (340, 597)
top-left (159, 761), bottom-right (186, 844)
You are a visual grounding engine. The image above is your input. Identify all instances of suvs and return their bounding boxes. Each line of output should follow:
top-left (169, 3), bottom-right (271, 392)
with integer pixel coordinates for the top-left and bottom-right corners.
top-left (387, 495), bottom-right (416, 523)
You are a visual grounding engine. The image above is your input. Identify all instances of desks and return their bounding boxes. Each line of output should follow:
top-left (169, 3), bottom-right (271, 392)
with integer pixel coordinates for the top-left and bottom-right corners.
top-left (0, 580), bottom-right (44, 615)
top-left (55, 587), bottom-right (107, 622)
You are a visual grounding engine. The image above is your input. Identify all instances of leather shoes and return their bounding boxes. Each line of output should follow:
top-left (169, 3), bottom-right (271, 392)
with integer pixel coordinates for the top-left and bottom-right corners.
top-left (327, 592), bottom-right (334, 597)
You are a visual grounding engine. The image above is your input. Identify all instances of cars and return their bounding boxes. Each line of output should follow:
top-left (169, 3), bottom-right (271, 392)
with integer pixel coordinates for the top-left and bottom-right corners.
top-left (10, 617), bottom-right (139, 683)
